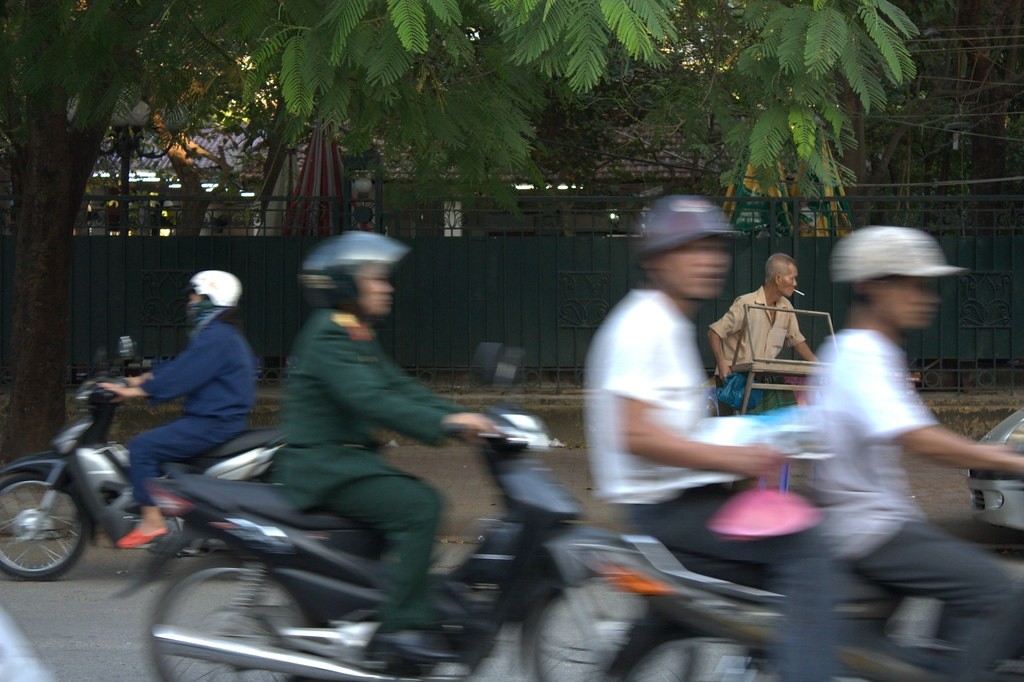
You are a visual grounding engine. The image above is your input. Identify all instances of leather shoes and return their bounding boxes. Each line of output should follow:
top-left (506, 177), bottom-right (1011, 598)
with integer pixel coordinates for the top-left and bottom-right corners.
top-left (361, 630), bottom-right (463, 662)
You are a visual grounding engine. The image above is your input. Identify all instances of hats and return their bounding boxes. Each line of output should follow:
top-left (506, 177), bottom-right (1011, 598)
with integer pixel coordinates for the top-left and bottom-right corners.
top-left (828, 224), bottom-right (972, 287)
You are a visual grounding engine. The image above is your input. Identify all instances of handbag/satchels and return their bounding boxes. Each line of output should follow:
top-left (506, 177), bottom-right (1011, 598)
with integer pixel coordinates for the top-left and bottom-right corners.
top-left (716, 371), bottom-right (763, 411)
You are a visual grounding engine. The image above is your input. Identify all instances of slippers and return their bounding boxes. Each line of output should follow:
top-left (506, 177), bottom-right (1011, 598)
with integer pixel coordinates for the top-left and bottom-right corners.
top-left (115, 528), bottom-right (169, 548)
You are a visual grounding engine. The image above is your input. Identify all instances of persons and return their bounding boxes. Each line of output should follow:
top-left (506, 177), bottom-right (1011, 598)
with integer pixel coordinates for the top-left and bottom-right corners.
top-left (95, 269), bottom-right (260, 549)
top-left (705, 252), bottom-right (819, 417)
top-left (577, 192), bottom-right (848, 682)
top-left (798, 226), bottom-right (1024, 681)
top-left (273, 228), bottom-right (509, 667)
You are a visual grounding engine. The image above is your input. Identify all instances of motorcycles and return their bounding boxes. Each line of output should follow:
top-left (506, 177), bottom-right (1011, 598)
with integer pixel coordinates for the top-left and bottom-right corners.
top-left (140, 402), bottom-right (690, 681)
top-left (3, 333), bottom-right (288, 583)
top-left (523, 409), bottom-right (1023, 682)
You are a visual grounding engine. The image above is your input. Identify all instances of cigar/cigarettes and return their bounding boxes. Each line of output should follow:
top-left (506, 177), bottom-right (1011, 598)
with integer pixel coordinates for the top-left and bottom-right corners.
top-left (793, 289), bottom-right (805, 296)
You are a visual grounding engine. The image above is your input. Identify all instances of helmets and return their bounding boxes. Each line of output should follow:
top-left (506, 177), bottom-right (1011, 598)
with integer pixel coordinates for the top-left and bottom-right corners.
top-left (297, 230), bottom-right (412, 323)
top-left (627, 187), bottom-right (755, 280)
top-left (189, 269), bottom-right (242, 307)
